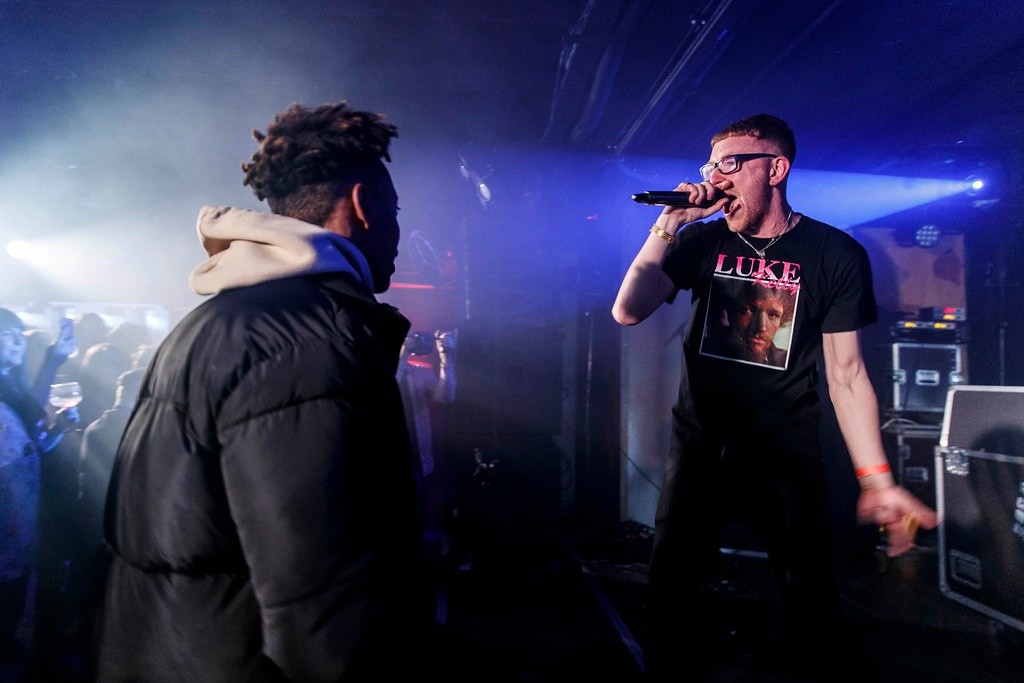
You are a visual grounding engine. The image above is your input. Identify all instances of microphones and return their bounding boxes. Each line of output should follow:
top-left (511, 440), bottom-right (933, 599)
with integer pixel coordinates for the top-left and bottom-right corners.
top-left (632, 186), bottom-right (728, 209)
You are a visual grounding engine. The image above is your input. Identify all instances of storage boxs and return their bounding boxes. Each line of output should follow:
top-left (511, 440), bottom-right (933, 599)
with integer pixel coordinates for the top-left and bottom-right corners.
top-left (877, 342), bottom-right (1024, 634)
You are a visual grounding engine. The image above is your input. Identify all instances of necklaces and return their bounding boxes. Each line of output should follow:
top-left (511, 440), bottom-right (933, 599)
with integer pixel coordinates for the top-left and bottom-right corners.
top-left (736, 210), bottom-right (791, 260)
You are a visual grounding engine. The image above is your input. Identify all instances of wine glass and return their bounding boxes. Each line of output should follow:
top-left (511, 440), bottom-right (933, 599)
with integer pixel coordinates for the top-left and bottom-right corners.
top-left (47, 381), bottom-right (84, 434)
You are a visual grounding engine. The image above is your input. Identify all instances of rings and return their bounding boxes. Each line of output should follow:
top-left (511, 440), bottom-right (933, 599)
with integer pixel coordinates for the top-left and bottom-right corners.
top-left (878, 526), bottom-right (885, 533)
top-left (686, 182), bottom-right (693, 190)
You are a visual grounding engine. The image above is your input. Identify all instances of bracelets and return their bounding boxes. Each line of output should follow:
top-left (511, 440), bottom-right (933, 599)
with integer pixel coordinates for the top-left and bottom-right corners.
top-left (649, 223), bottom-right (676, 245)
top-left (855, 462), bottom-right (891, 480)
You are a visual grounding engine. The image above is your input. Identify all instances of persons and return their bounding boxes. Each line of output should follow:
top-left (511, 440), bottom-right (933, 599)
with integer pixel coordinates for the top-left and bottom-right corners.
top-left (95, 96), bottom-right (427, 683)
top-left (609, 111), bottom-right (939, 683)
top-left (0, 295), bottom-right (455, 683)
top-left (701, 280), bottom-right (798, 369)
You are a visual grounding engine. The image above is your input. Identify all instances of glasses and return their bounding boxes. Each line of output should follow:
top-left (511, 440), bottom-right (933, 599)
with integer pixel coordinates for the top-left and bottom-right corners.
top-left (699, 153), bottom-right (777, 183)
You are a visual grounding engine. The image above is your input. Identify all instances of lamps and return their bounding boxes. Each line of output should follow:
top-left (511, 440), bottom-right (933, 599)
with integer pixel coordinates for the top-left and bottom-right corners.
top-left (461, 182), bottom-right (491, 207)
